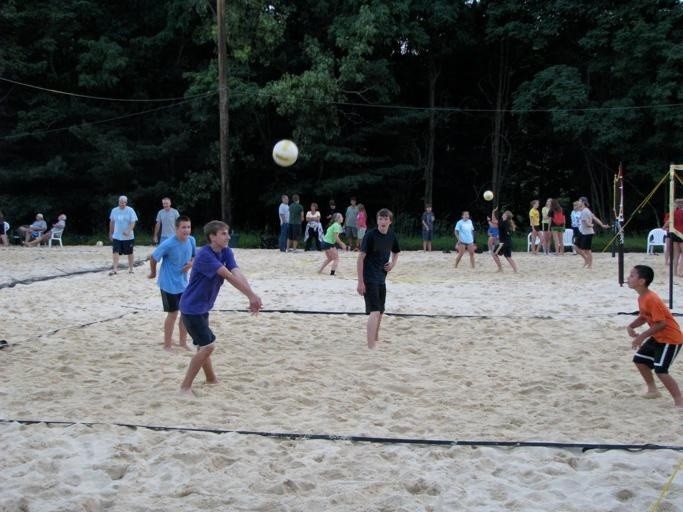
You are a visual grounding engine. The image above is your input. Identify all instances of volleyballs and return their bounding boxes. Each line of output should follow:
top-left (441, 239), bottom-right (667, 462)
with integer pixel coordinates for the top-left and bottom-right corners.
top-left (271, 140), bottom-right (299, 167)
top-left (483, 190), bottom-right (494, 201)
top-left (97, 241), bottom-right (103, 247)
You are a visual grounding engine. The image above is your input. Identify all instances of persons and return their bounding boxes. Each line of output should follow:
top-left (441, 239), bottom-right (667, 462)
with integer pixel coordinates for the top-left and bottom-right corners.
top-left (356, 208), bottom-right (401, 349)
top-left (304, 203), bottom-right (322, 252)
top-left (548, 200), bottom-right (566, 256)
top-left (279, 195), bottom-right (290, 252)
top-left (285, 194), bottom-right (304, 253)
top-left (663, 200), bottom-right (683, 279)
top-left (147, 215), bottom-right (197, 352)
top-left (454, 211), bottom-right (476, 269)
top-left (325, 200), bottom-right (340, 222)
top-left (529, 200), bottom-right (542, 254)
top-left (421, 204), bottom-right (435, 253)
top-left (491, 206), bottom-right (517, 274)
top-left (0, 212), bottom-right (8, 246)
top-left (23, 214), bottom-right (67, 247)
top-left (108, 196), bottom-right (138, 276)
top-left (625, 264), bottom-right (683, 408)
top-left (487, 216), bottom-right (499, 254)
top-left (542, 198), bottom-right (552, 256)
top-left (180, 220), bottom-right (263, 392)
top-left (25, 213), bottom-right (47, 247)
top-left (663, 212), bottom-right (671, 264)
top-left (344, 199), bottom-right (358, 246)
top-left (355, 204), bottom-right (367, 250)
top-left (575, 196), bottom-right (610, 270)
top-left (153, 198), bottom-right (180, 246)
top-left (317, 212), bottom-right (346, 276)
top-left (571, 201), bottom-right (582, 254)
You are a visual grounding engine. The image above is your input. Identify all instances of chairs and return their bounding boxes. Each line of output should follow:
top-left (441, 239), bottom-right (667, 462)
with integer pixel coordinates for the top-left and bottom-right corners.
top-left (561, 230), bottom-right (577, 253)
top-left (530, 231), bottom-right (545, 253)
top-left (51, 229), bottom-right (63, 246)
top-left (646, 228), bottom-right (668, 256)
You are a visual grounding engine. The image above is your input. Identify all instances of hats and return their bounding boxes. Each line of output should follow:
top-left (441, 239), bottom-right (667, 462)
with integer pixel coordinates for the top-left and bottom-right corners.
top-left (580, 197), bottom-right (590, 207)
